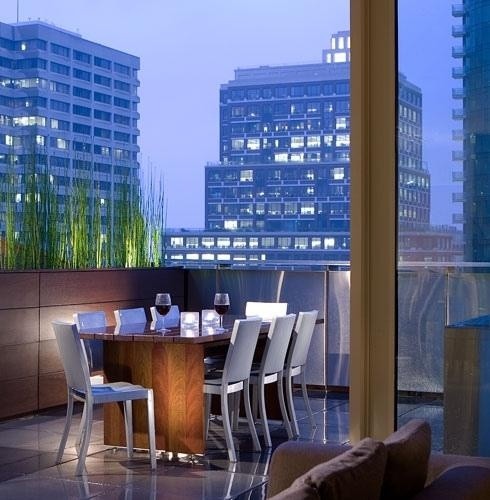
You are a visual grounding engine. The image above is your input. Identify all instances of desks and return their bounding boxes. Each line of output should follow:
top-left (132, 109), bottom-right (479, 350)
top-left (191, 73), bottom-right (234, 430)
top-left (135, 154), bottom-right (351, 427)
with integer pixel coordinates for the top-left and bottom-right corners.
top-left (77, 318), bottom-right (292, 456)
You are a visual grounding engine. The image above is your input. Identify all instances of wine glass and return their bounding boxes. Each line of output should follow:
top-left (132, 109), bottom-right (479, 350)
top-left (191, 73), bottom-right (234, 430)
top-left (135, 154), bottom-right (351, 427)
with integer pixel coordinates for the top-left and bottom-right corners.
top-left (214, 293), bottom-right (230, 331)
top-left (155, 293), bottom-right (172, 334)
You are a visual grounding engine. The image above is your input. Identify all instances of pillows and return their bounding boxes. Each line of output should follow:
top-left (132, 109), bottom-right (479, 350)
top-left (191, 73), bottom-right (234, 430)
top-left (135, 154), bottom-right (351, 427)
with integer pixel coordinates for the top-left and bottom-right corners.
top-left (267, 436), bottom-right (388, 499)
top-left (382, 418), bottom-right (432, 499)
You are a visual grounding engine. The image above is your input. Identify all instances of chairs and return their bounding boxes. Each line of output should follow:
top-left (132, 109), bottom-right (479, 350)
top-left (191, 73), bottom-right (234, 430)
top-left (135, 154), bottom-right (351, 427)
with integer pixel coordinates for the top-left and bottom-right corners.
top-left (150, 306), bottom-right (178, 319)
top-left (52, 322), bottom-right (157, 476)
top-left (73, 310), bottom-right (106, 447)
top-left (204, 317), bottom-right (264, 462)
top-left (113, 307), bottom-right (146, 325)
top-left (280, 310), bottom-right (319, 436)
top-left (234, 315), bottom-right (296, 446)
top-left (245, 302), bottom-right (288, 322)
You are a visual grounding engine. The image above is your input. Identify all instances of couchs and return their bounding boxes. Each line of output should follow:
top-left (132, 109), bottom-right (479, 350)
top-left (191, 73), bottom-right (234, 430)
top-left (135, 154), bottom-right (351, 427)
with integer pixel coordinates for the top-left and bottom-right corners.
top-left (266, 442), bottom-right (490, 500)
